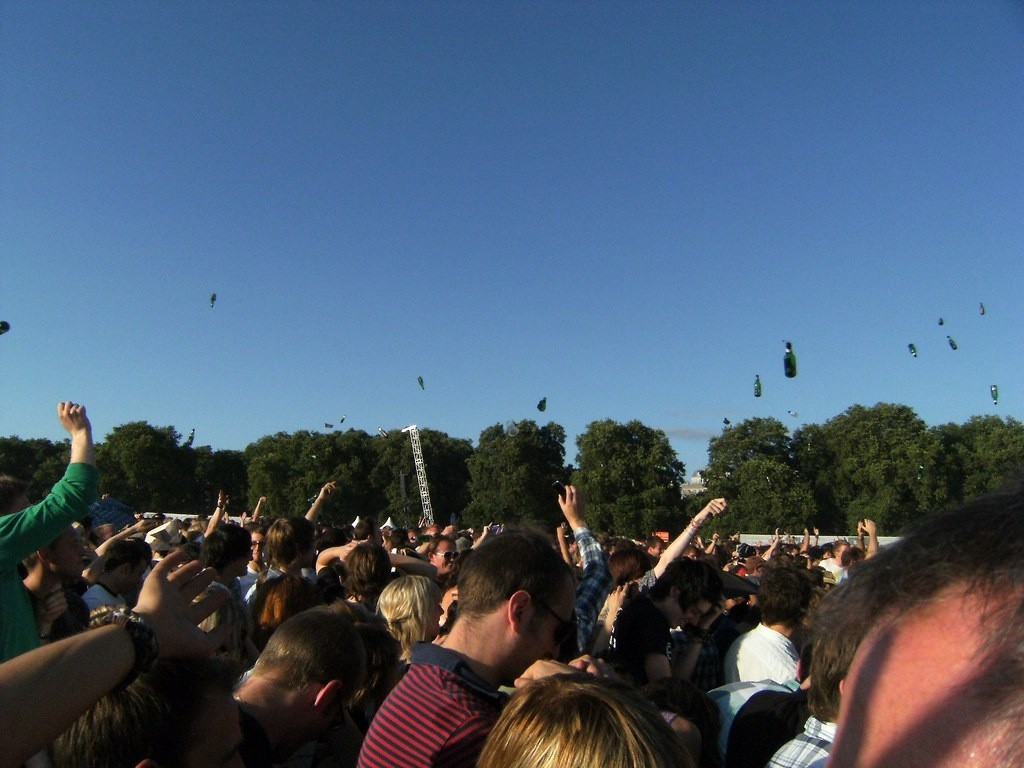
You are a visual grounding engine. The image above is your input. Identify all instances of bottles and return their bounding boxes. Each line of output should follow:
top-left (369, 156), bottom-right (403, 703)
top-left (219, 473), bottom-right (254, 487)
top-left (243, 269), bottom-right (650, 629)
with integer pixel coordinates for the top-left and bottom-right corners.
top-left (908, 344), bottom-right (917, 357)
top-left (340, 415), bottom-right (346, 423)
top-left (417, 376), bottom-right (425, 390)
top-left (324, 423), bottom-right (333, 428)
top-left (990, 385), bottom-right (998, 405)
top-left (211, 293), bottom-right (216, 307)
top-left (788, 411), bottom-right (799, 417)
top-left (378, 427), bottom-right (388, 438)
top-left (783, 342), bottom-right (796, 378)
top-left (979, 303), bottom-right (985, 315)
top-left (754, 375), bottom-right (762, 397)
top-left (947, 336), bottom-right (957, 350)
top-left (917, 464), bottom-right (925, 481)
top-left (536, 397), bottom-right (548, 412)
top-left (939, 318), bottom-right (944, 325)
top-left (724, 418), bottom-right (730, 425)
top-left (0, 321), bottom-right (9, 335)
top-left (188, 429), bottom-right (195, 446)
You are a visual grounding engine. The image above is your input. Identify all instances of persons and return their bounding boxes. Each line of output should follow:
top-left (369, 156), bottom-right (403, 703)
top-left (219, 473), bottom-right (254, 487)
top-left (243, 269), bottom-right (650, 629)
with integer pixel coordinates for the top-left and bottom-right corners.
top-left (0, 403), bottom-right (1024, 768)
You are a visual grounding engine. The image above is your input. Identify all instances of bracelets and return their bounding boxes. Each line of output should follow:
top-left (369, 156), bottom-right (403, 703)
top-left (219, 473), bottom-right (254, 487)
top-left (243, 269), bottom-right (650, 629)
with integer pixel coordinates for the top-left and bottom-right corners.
top-left (684, 529), bottom-right (694, 540)
top-left (217, 506), bottom-right (223, 509)
top-left (102, 607), bottom-right (159, 666)
top-left (689, 625), bottom-right (708, 644)
top-left (686, 525), bottom-right (698, 533)
top-left (691, 519), bottom-right (703, 529)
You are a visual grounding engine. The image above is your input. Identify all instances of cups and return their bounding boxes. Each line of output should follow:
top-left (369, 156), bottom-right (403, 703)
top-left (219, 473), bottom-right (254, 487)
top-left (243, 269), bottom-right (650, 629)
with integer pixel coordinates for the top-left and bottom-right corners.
top-left (505, 419), bottom-right (519, 437)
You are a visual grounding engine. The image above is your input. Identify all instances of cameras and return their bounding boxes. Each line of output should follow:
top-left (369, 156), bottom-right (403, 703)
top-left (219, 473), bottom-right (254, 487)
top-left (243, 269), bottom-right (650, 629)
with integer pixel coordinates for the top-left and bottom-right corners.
top-left (860, 521), bottom-right (867, 532)
top-left (488, 523), bottom-right (501, 532)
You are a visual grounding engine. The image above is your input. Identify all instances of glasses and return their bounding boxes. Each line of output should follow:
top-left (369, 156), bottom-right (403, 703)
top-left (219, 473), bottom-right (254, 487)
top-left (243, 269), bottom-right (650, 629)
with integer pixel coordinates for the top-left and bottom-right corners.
top-left (506, 590), bottom-right (575, 646)
top-left (251, 541), bottom-right (263, 546)
top-left (434, 549), bottom-right (459, 559)
top-left (409, 536), bottom-right (416, 540)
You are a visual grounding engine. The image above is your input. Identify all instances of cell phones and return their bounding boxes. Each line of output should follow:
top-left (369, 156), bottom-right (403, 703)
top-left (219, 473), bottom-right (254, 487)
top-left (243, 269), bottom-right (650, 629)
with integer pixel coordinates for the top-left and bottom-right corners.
top-left (551, 480), bottom-right (566, 496)
